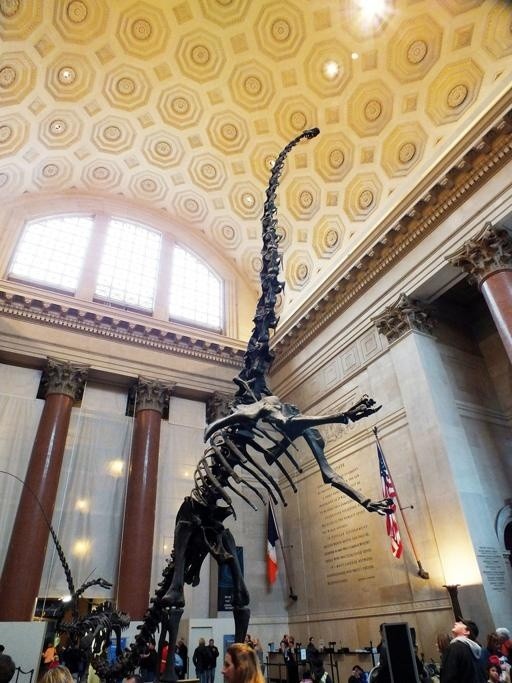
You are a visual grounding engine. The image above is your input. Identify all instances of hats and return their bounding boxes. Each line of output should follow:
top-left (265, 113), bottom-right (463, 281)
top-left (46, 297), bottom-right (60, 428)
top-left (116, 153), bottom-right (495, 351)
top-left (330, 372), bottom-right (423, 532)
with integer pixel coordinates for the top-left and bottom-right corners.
top-left (459, 616), bottom-right (479, 639)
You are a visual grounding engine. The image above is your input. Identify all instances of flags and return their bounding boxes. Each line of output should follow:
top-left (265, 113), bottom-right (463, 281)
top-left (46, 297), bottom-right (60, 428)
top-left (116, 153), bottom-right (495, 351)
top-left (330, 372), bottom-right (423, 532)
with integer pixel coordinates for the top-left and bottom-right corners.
top-left (376, 437), bottom-right (404, 563)
top-left (266, 502), bottom-right (279, 591)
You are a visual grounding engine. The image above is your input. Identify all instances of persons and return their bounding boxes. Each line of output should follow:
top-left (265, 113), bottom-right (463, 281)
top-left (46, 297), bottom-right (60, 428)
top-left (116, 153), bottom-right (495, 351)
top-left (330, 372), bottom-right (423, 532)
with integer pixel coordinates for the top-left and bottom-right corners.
top-left (376, 615), bottom-right (511, 682)
top-left (280, 635), bottom-right (367, 682)
top-left (193, 634), bottom-right (265, 682)
top-left (40, 638), bottom-right (188, 681)
top-left (0, 645), bottom-right (15, 683)
